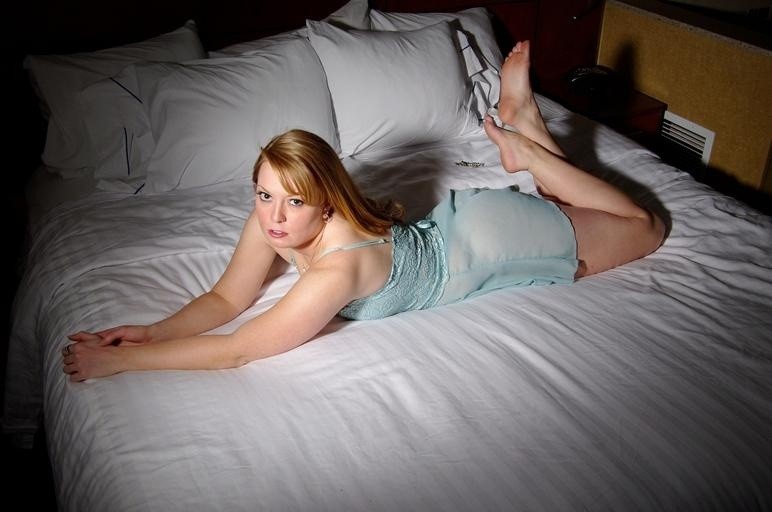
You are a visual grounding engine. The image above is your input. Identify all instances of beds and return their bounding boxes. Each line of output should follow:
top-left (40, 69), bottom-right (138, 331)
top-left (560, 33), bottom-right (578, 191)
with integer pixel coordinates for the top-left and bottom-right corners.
top-left (9, 91), bottom-right (770, 511)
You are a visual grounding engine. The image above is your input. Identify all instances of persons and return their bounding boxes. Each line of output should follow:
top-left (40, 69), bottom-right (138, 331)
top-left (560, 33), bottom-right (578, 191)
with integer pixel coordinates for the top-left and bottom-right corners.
top-left (61, 39), bottom-right (666, 382)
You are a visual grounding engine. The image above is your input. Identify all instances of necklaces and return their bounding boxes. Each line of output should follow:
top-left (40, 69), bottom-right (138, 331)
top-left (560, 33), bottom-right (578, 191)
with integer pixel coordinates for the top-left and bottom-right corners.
top-left (297, 226), bottom-right (325, 274)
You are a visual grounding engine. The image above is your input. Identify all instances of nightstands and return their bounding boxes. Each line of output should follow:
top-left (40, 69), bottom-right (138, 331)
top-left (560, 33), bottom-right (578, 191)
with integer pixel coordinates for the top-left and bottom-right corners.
top-left (534, 71), bottom-right (669, 148)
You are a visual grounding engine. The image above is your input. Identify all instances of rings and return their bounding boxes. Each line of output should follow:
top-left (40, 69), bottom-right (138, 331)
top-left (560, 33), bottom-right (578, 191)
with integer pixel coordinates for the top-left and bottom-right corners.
top-left (65, 345), bottom-right (72, 355)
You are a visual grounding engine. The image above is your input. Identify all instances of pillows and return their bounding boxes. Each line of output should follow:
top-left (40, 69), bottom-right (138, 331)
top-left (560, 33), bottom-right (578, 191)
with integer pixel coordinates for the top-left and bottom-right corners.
top-left (25, 19), bottom-right (205, 183)
top-left (366, 5), bottom-right (506, 109)
top-left (126, 36), bottom-right (343, 189)
top-left (205, 1), bottom-right (369, 60)
top-left (304, 17), bottom-right (482, 156)
